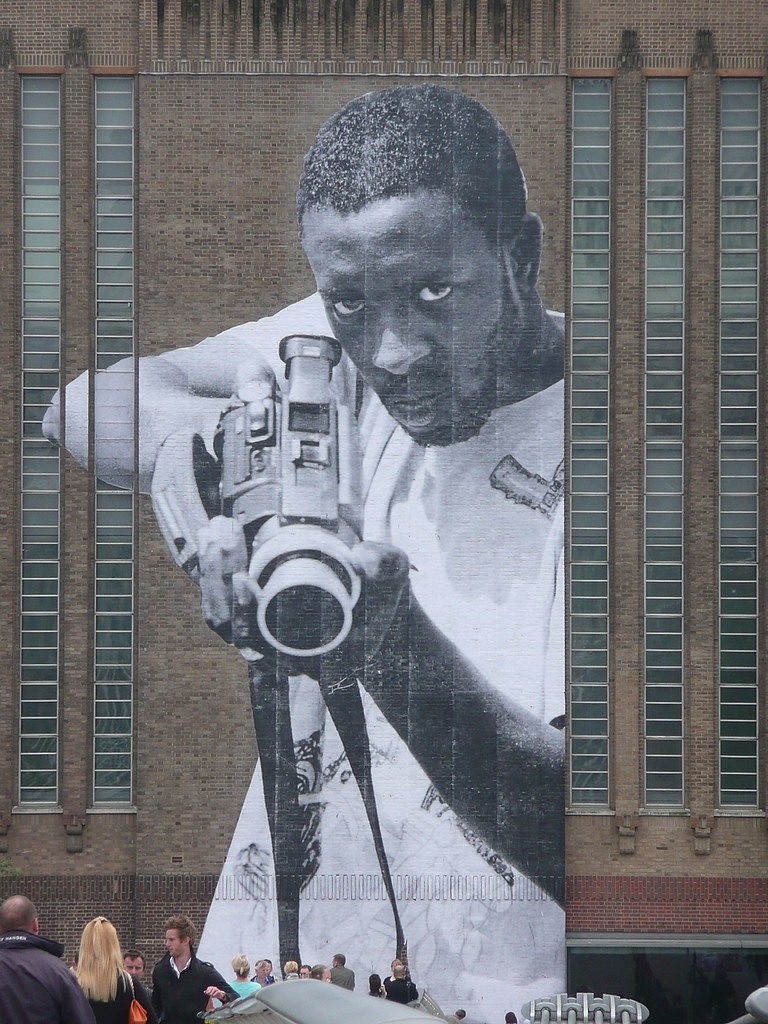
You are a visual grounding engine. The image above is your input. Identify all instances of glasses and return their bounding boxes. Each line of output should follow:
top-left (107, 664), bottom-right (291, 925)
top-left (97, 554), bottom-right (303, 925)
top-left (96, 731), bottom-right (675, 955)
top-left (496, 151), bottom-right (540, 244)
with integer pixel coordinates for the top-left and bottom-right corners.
top-left (299, 972), bottom-right (310, 976)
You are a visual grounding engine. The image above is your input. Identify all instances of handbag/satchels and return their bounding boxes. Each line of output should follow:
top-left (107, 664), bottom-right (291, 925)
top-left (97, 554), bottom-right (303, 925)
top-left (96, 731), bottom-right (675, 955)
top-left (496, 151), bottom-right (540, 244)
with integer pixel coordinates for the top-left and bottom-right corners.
top-left (122, 969), bottom-right (148, 1024)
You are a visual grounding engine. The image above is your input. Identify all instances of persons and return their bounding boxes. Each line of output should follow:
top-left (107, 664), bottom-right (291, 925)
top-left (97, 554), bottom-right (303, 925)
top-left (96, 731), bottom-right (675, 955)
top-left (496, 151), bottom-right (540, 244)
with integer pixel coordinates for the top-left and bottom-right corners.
top-left (0, 894), bottom-right (97, 1024)
top-left (64, 916), bottom-right (518, 1023)
top-left (41, 84), bottom-right (567, 1023)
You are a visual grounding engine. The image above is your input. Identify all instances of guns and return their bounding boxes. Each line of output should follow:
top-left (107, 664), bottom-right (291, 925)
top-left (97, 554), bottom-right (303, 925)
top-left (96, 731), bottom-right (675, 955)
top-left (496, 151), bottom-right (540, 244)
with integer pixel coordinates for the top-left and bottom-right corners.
top-left (211, 329), bottom-right (367, 664)
